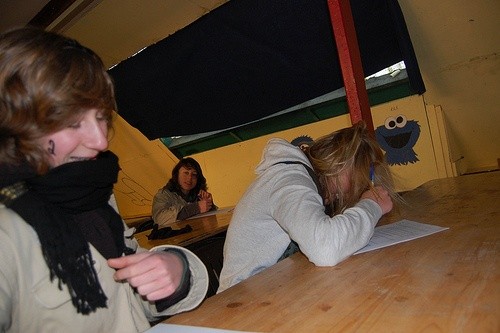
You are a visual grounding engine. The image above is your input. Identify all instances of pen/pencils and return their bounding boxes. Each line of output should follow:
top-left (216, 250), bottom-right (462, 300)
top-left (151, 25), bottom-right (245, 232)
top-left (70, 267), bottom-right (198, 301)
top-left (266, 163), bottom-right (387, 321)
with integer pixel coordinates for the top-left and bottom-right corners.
top-left (197, 195), bottom-right (203, 201)
top-left (370, 165), bottom-right (375, 189)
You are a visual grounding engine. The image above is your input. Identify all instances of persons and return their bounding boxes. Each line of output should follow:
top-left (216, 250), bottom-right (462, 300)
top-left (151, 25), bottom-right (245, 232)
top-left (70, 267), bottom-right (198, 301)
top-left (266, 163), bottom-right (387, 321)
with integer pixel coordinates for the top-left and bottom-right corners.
top-left (151, 156), bottom-right (218, 230)
top-left (210, 123), bottom-right (412, 295)
top-left (0, 23), bottom-right (210, 333)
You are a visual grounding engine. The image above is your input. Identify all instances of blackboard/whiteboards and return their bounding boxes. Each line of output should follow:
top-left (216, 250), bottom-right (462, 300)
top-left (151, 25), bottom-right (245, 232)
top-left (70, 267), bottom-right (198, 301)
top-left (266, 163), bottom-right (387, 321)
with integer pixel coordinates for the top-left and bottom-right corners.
top-left (105, 0), bottom-right (427, 158)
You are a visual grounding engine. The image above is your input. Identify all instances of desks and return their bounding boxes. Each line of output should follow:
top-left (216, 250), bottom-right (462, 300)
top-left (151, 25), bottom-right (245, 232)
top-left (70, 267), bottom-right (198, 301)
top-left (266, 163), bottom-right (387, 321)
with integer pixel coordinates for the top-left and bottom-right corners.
top-left (122, 205), bottom-right (236, 265)
top-left (145, 170), bottom-right (500, 333)
